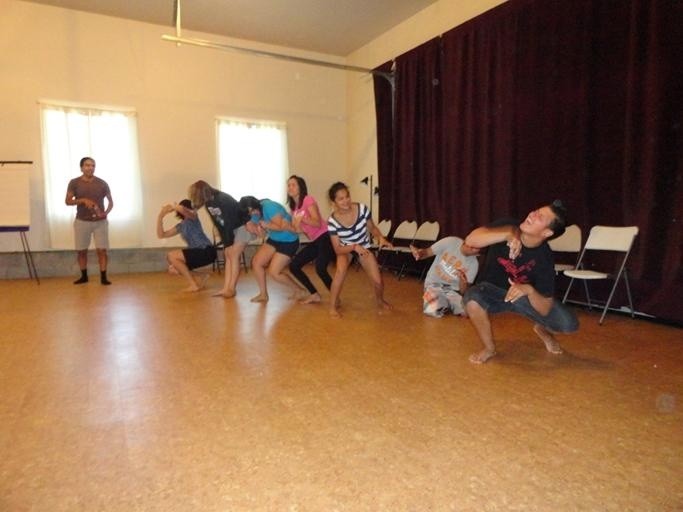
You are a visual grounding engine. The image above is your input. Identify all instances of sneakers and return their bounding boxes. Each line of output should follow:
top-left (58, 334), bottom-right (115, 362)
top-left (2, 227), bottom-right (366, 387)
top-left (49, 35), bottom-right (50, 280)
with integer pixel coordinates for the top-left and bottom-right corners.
top-left (101, 279), bottom-right (110, 285)
top-left (74, 276), bottom-right (88, 284)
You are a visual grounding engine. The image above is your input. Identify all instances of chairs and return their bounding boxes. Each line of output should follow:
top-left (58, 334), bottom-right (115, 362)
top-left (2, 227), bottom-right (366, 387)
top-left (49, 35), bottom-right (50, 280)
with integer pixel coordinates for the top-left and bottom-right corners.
top-left (369, 219), bottom-right (440, 284)
top-left (562, 225), bottom-right (639, 326)
top-left (546, 224), bottom-right (592, 312)
top-left (212, 225), bottom-right (247, 275)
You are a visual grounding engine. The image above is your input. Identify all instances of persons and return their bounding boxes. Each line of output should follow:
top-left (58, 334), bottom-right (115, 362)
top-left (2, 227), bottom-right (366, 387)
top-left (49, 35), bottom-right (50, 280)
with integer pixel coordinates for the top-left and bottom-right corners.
top-left (65, 157), bottom-right (113, 285)
top-left (188, 180), bottom-right (257, 298)
top-left (238, 196), bottom-right (306, 303)
top-left (282, 175), bottom-right (342, 305)
top-left (463, 199), bottom-right (580, 364)
top-left (158, 198), bottom-right (217, 293)
top-left (327, 182), bottom-right (394, 320)
top-left (410, 236), bottom-right (488, 318)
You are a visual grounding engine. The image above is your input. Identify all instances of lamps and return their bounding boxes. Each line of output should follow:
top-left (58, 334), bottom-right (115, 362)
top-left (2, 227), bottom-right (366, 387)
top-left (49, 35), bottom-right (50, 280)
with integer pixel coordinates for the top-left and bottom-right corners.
top-left (360, 175), bottom-right (379, 212)
